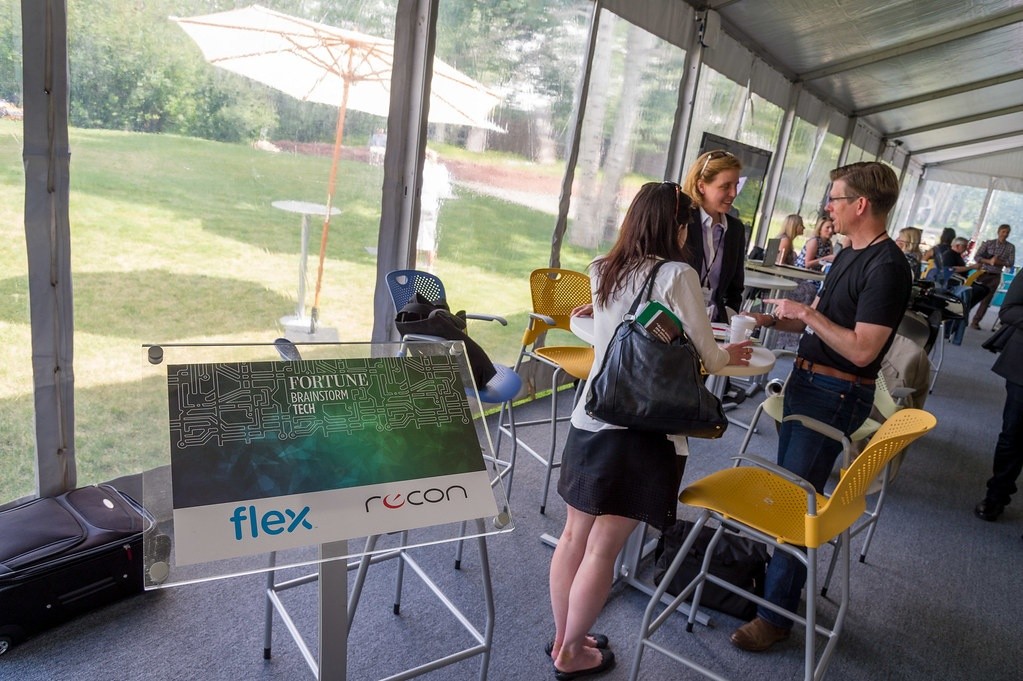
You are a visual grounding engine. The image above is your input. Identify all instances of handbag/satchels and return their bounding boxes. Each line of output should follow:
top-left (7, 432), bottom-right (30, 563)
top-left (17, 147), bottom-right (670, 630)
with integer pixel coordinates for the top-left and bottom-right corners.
top-left (582, 260), bottom-right (728, 439)
top-left (653, 520), bottom-right (772, 622)
top-left (394, 290), bottom-right (497, 393)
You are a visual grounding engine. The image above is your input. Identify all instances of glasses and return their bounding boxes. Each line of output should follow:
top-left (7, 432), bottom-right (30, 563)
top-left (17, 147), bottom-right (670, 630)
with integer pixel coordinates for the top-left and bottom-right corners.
top-left (641, 179), bottom-right (682, 224)
top-left (821, 216), bottom-right (831, 220)
top-left (699, 151), bottom-right (737, 176)
top-left (826, 195), bottom-right (870, 205)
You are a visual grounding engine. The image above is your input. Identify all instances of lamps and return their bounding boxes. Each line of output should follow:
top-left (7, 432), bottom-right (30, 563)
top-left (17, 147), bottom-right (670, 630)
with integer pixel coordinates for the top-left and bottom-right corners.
top-left (886, 140), bottom-right (904, 148)
top-left (695, 11), bottom-right (703, 22)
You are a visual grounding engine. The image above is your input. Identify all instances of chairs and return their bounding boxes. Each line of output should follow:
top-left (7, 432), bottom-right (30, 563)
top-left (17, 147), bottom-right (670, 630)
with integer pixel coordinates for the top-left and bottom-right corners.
top-left (929, 268), bottom-right (988, 330)
top-left (494, 268), bottom-right (593, 514)
top-left (729, 333), bottom-right (929, 598)
top-left (386, 269), bottom-right (522, 571)
top-left (274, 338), bottom-right (305, 362)
top-left (631, 408), bottom-right (938, 681)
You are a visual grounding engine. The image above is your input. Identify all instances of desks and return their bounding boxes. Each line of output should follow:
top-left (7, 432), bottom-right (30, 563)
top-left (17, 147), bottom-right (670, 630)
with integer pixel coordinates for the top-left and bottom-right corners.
top-left (705, 269), bottom-right (798, 434)
top-left (539, 313), bottom-right (774, 626)
top-left (730, 258), bottom-right (829, 398)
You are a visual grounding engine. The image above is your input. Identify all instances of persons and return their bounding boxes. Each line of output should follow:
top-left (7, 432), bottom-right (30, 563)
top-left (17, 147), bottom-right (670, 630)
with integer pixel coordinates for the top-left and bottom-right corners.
top-left (775, 211), bottom-right (1015, 345)
top-left (733, 161), bottom-right (912, 651)
top-left (655, 149), bottom-right (749, 533)
top-left (546, 181), bottom-right (757, 677)
top-left (978, 268), bottom-right (1023, 521)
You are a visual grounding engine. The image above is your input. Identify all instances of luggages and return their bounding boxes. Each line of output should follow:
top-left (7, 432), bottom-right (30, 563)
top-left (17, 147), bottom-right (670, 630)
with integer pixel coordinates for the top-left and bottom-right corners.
top-left (0, 483), bottom-right (172, 656)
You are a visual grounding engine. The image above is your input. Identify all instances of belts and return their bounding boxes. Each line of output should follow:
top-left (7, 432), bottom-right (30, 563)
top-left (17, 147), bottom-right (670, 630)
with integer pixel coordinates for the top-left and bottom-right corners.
top-left (794, 356), bottom-right (876, 386)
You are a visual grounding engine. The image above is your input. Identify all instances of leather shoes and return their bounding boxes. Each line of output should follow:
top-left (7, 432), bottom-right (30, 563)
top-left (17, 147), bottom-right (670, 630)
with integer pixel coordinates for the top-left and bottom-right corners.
top-left (974, 498), bottom-right (1005, 522)
top-left (729, 616), bottom-right (790, 653)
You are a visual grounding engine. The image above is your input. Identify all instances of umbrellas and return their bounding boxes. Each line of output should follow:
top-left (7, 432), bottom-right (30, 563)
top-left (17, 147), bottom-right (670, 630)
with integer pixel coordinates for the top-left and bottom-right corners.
top-left (169, 4), bottom-right (507, 332)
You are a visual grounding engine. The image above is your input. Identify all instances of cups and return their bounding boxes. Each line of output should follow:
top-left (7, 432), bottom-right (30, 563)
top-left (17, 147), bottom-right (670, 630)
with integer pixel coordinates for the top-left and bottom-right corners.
top-left (730, 314), bottom-right (757, 343)
top-left (722, 326), bottom-right (753, 348)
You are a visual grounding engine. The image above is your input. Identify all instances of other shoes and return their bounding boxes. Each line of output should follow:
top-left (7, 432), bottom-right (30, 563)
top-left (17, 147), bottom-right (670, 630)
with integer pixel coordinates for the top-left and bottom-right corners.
top-left (554, 647), bottom-right (615, 679)
top-left (545, 631), bottom-right (609, 657)
top-left (970, 322), bottom-right (980, 330)
